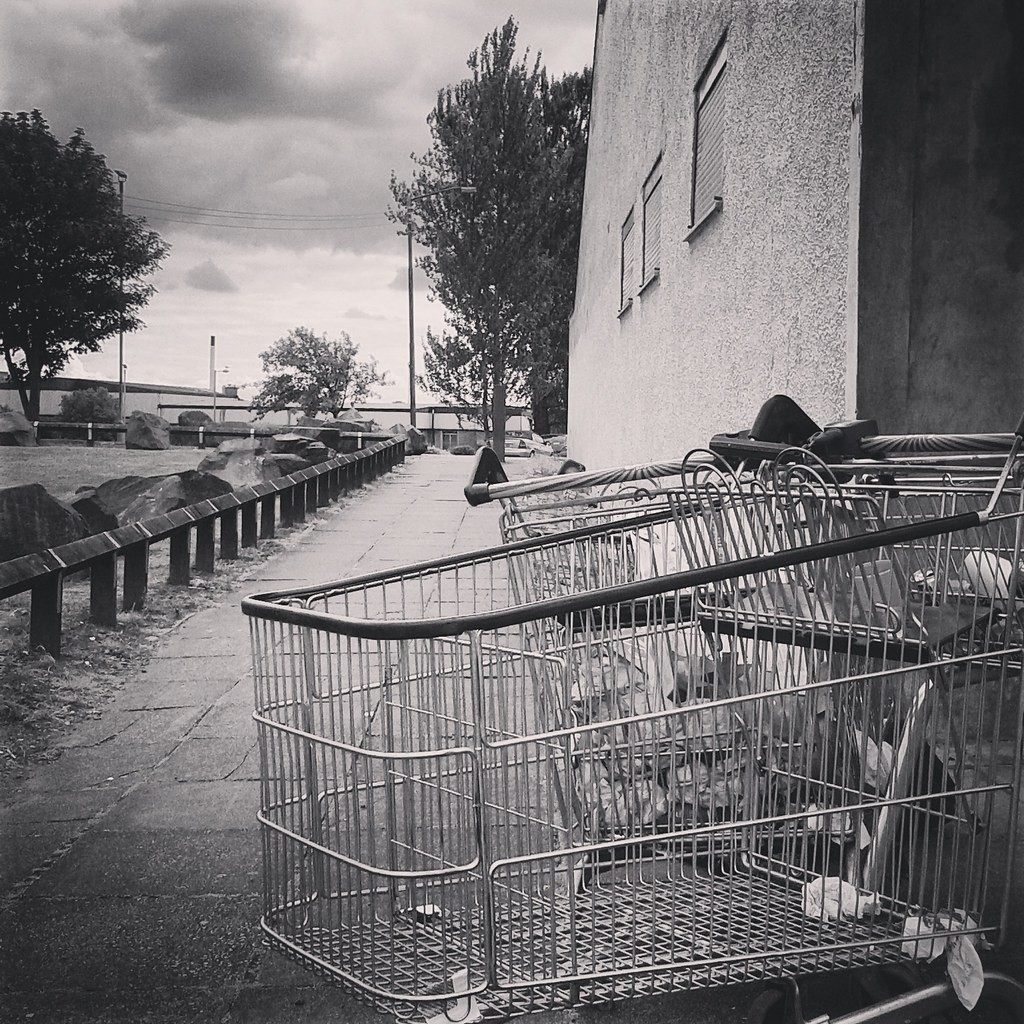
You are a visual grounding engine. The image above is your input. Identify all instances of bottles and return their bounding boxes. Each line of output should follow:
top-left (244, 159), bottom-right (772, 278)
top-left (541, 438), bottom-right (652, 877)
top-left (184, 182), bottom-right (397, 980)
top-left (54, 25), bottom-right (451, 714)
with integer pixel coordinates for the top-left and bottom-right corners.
top-left (908, 564), bottom-right (950, 605)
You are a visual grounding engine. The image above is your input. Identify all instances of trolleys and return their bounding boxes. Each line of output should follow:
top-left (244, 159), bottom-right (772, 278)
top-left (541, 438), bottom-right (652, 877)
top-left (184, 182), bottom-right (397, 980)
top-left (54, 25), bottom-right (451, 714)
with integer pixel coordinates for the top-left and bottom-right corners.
top-left (240, 394), bottom-right (1024, 1023)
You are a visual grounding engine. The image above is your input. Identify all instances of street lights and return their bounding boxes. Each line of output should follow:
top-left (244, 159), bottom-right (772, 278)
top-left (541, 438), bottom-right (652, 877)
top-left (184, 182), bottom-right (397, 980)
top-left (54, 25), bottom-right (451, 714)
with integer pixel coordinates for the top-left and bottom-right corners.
top-left (114, 169), bottom-right (127, 419)
top-left (407, 185), bottom-right (478, 428)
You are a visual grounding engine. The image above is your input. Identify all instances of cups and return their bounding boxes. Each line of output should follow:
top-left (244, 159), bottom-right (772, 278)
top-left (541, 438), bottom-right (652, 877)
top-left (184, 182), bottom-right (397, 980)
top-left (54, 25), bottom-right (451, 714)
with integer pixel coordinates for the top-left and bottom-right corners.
top-left (846, 559), bottom-right (904, 611)
top-left (957, 546), bottom-right (1024, 615)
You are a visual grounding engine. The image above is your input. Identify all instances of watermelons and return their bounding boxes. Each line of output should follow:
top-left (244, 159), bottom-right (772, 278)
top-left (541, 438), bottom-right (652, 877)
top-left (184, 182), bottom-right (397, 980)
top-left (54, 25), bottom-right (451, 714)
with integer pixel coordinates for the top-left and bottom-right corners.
top-left (561, 651), bottom-right (811, 842)
